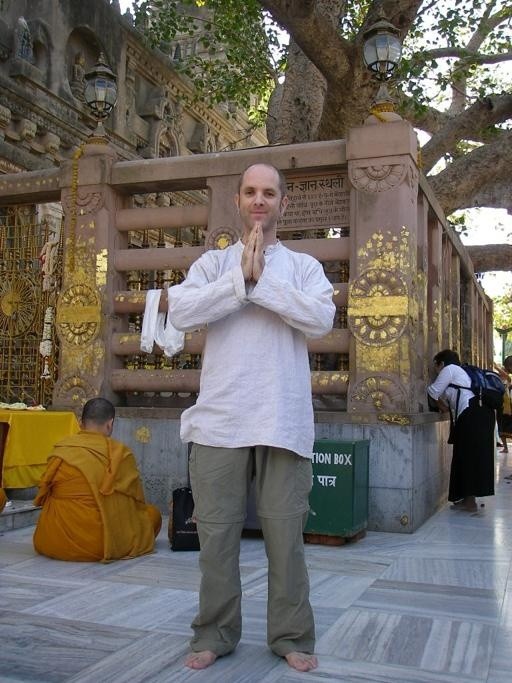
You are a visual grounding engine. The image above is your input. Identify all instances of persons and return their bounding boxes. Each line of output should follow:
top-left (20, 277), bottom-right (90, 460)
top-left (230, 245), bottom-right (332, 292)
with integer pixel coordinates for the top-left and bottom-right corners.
top-left (32, 396), bottom-right (162, 564)
top-left (424, 347), bottom-right (511, 514)
top-left (168, 161), bottom-right (337, 673)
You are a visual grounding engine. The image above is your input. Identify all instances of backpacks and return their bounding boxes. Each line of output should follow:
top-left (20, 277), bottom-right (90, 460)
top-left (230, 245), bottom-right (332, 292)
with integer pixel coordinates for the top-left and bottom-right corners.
top-left (449, 364), bottom-right (505, 409)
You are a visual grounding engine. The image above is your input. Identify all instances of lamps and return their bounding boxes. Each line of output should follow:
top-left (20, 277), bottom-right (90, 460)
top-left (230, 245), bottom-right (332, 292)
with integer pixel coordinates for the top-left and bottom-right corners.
top-left (82, 52), bottom-right (118, 142)
top-left (362, 5), bottom-right (402, 105)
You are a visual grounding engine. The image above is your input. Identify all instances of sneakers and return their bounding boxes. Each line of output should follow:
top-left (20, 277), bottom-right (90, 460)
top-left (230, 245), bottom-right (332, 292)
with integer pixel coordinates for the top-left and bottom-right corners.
top-left (449, 498), bottom-right (477, 511)
top-left (500, 448), bottom-right (508, 453)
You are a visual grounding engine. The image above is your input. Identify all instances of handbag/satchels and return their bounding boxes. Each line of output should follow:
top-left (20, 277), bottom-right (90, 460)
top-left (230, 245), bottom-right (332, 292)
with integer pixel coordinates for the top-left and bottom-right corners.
top-left (167, 486), bottom-right (200, 551)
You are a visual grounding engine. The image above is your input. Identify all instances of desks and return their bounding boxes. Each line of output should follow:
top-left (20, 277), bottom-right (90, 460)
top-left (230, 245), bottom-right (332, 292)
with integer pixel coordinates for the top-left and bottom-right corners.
top-left (0, 408), bottom-right (74, 489)
